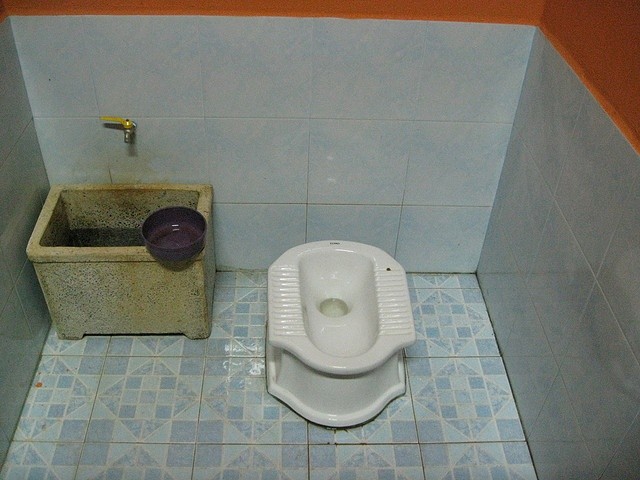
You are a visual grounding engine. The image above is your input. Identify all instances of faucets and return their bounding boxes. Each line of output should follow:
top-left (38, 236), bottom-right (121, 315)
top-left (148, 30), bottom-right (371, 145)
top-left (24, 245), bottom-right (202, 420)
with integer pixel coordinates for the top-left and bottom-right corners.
top-left (99, 116), bottom-right (136, 143)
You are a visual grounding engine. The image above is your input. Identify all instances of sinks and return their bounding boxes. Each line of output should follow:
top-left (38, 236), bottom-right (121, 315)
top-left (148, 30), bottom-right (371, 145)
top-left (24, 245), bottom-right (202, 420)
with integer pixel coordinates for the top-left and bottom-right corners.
top-left (25, 183), bottom-right (218, 339)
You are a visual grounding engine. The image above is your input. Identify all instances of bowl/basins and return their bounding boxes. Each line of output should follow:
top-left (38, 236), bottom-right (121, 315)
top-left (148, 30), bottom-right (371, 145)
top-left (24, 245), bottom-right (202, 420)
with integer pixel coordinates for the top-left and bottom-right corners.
top-left (141, 205), bottom-right (207, 262)
